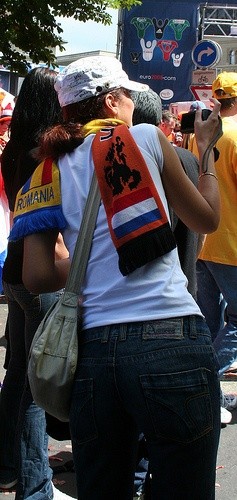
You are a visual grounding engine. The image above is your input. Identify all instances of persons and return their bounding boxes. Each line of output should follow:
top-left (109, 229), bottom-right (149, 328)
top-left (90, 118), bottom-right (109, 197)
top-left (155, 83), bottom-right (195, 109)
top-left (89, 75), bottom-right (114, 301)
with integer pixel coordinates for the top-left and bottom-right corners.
top-left (0, 90), bottom-right (209, 302)
top-left (0, 68), bottom-right (76, 500)
top-left (187, 70), bottom-right (237, 423)
top-left (7, 55), bottom-right (224, 500)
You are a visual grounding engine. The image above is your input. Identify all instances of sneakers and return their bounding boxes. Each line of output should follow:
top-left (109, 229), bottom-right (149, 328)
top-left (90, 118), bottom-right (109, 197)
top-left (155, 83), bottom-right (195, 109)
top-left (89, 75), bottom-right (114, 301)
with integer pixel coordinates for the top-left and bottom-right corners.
top-left (223, 362), bottom-right (237, 376)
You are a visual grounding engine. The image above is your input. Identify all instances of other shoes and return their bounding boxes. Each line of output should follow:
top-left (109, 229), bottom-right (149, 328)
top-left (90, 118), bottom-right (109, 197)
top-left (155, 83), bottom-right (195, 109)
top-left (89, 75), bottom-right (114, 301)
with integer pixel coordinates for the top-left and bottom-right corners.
top-left (221, 393), bottom-right (237, 424)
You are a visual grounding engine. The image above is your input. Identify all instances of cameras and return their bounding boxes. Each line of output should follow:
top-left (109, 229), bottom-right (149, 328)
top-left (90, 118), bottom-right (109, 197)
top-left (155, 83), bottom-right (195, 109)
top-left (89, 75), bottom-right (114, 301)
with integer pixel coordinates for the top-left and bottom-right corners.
top-left (180, 109), bottom-right (221, 134)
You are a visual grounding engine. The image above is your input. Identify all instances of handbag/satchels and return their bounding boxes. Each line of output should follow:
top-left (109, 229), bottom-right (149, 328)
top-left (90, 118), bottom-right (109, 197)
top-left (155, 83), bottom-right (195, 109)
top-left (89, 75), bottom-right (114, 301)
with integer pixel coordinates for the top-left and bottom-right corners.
top-left (27, 294), bottom-right (78, 423)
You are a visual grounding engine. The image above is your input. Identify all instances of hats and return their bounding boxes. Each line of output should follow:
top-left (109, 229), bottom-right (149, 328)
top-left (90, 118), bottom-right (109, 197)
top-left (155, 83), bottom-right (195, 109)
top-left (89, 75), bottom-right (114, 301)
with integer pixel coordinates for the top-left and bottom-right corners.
top-left (53, 55), bottom-right (149, 108)
top-left (212, 72), bottom-right (237, 99)
top-left (190, 101), bottom-right (209, 111)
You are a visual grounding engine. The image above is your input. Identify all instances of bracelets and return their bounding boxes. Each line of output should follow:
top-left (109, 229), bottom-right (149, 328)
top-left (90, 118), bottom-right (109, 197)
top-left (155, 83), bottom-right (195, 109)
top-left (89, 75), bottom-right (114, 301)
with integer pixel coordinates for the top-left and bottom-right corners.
top-left (199, 172), bottom-right (218, 180)
